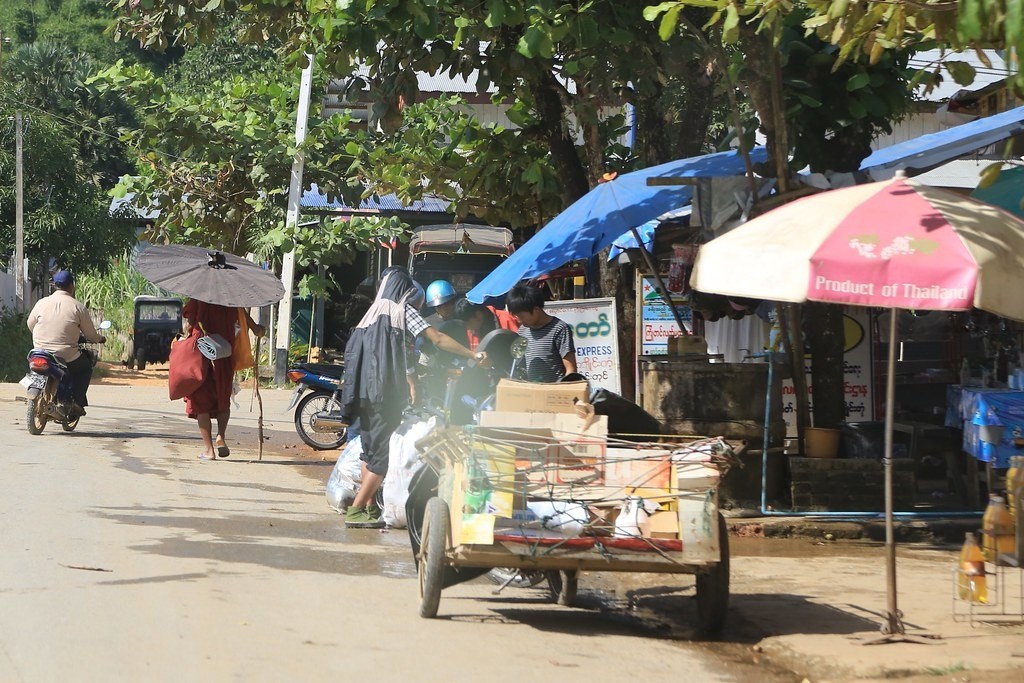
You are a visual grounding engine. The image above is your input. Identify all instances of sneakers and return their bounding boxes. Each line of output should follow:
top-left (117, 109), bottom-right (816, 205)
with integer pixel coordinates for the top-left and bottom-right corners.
top-left (366, 505), bottom-right (382, 519)
top-left (74, 403), bottom-right (86, 416)
top-left (345, 506), bottom-right (386, 528)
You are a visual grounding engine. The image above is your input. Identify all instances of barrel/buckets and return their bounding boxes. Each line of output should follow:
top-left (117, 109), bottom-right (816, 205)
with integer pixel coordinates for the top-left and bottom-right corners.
top-left (668, 334), bottom-right (708, 363)
top-left (806, 427), bottom-right (841, 458)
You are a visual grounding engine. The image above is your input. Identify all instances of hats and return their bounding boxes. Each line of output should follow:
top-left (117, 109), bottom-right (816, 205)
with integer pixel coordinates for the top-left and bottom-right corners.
top-left (53, 271), bottom-right (72, 288)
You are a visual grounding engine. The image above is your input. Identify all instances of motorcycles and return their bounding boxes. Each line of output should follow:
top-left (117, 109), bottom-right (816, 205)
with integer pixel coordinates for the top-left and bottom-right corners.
top-left (285, 362), bottom-right (349, 451)
top-left (22, 321), bottom-right (111, 435)
top-left (405, 329), bottom-right (730, 641)
top-left (134, 296), bottom-right (183, 370)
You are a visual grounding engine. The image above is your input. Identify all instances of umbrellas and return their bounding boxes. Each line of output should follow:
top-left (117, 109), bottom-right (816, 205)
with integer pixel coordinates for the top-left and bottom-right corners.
top-left (135, 244), bottom-right (286, 307)
top-left (464, 145), bottom-right (768, 334)
top-left (686, 170), bottom-right (1024, 612)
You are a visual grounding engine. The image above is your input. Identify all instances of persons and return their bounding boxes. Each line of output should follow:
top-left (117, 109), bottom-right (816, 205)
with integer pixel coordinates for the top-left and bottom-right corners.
top-left (506, 284), bottom-right (578, 383)
top-left (453, 297), bottom-right (522, 352)
top-left (340, 269), bottom-right (492, 529)
top-left (27, 271), bottom-right (106, 416)
top-left (419, 279), bottom-right (462, 364)
top-left (182, 298), bottom-right (265, 460)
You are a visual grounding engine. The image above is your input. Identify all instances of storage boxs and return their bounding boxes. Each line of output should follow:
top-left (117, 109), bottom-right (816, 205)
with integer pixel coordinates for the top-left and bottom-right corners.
top-left (434, 378), bottom-right (720, 565)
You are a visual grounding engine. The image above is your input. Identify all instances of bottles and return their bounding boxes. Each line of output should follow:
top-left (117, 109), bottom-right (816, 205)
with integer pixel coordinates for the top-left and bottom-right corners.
top-left (981, 456), bottom-right (1024, 565)
top-left (764, 306), bottom-right (793, 353)
top-left (614, 494), bottom-right (652, 539)
top-left (957, 532), bottom-right (987, 603)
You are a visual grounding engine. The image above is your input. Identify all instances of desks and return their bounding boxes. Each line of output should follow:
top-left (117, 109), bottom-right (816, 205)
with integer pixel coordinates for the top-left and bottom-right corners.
top-left (944, 383), bottom-right (1024, 507)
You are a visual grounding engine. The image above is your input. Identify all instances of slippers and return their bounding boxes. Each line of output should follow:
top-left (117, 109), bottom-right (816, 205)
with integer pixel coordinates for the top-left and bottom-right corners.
top-left (197, 452), bottom-right (213, 461)
top-left (217, 444), bottom-right (230, 457)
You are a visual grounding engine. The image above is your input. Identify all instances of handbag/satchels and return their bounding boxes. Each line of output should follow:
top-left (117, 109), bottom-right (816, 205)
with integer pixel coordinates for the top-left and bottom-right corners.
top-left (235, 307), bottom-right (254, 371)
top-left (197, 333), bottom-right (232, 360)
top-left (168, 330), bottom-right (208, 398)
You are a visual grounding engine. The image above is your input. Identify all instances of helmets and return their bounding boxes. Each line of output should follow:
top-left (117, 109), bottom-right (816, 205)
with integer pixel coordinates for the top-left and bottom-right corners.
top-left (426, 280), bottom-right (457, 307)
top-left (381, 264), bottom-right (411, 281)
